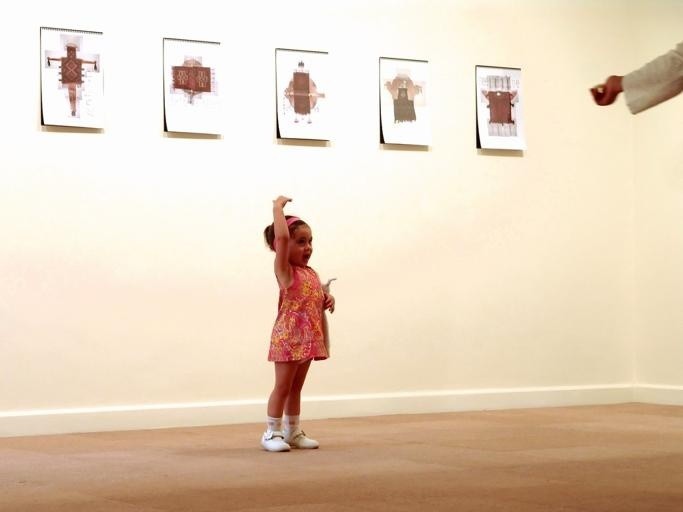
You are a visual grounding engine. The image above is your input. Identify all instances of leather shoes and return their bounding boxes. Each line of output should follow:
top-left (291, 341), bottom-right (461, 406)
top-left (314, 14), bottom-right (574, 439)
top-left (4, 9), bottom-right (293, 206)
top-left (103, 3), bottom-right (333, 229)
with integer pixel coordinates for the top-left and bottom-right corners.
top-left (285, 430), bottom-right (318, 449)
top-left (261, 431), bottom-right (288, 452)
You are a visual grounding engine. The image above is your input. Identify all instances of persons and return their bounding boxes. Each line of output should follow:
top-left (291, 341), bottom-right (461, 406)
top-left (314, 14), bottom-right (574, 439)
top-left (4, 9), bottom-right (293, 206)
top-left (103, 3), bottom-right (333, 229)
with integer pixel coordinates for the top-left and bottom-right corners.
top-left (588, 44), bottom-right (682, 113)
top-left (260, 194), bottom-right (335, 452)
